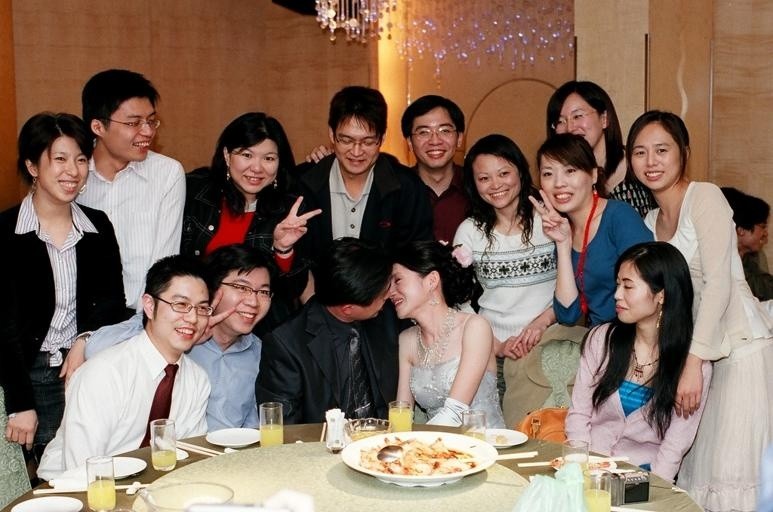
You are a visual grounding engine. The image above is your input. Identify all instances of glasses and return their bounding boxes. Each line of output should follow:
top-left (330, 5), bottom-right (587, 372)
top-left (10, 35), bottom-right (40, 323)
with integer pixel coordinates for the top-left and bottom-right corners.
top-left (220, 282), bottom-right (274, 300)
top-left (152, 296), bottom-right (213, 316)
top-left (410, 128), bottom-right (459, 138)
top-left (109, 119), bottom-right (161, 131)
top-left (334, 132), bottom-right (381, 148)
top-left (551, 112), bottom-right (598, 129)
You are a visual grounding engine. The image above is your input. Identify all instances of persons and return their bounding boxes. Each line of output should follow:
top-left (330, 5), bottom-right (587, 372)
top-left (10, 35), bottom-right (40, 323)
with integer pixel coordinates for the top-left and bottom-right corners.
top-left (255, 237), bottom-right (393, 426)
top-left (298, 85), bottom-right (434, 266)
top-left (547, 80), bottom-right (653, 220)
top-left (1, 112), bottom-right (127, 491)
top-left (84, 244), bottom-right (274, 430)
top-left (729, 195), bottom-right (771, 303)
top-left (720, 187), bottom-right (746, 203)
top-left (76, 69), bottom-right (186, 317)
top-left (180, 112), bottom-right (322, 338)
top-left (305, 94), bottom-right (464, 312)
top-left (564, 241), bottom-right (712, 485)
top-left (529, 134), bottom-right (654, 330)
top-left (626, 110), bottom-right (771, 510)
top-left (452, 134), bottom-right (556, 412)
top-left (387, 237), bottom-right (506, 430)
top-left (37, 255), bottom-right (211, 487)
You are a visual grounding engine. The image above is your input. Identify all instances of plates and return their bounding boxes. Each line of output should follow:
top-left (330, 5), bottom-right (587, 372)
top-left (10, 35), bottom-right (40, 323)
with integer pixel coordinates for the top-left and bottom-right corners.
top-left (341, 430), bottom-right (499, 488)
top-left (82, 457), bottom-right (147, 480)
top-left (10, 496), bottom-right (84, 512)
top-left (556, 452), bottom-right (617, 474)
top-left (206, 428), bottom-right (263, 447)
top-left (467, 428), bottom-right (530, 448)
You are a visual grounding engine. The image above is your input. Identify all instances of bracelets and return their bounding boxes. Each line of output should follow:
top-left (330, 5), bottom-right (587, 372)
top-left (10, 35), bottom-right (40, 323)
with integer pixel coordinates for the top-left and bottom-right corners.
top-left (272, 245), bottom-right (292, 254)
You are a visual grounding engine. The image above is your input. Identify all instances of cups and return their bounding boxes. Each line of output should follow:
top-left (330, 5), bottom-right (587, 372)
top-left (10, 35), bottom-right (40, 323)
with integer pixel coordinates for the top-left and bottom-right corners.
top-left (583, 468), bottom-right (615, 512)
top-left (388, 401), bottom-right (412, 432)
top-left (461, 409), bottom-right (487, 441)
top-left (149, 419), bottom-right (176, 471)
top-left (86, 456), bottom-right (116, 512)
top-left (259, 402), bottom-right (285, 447)
top-left (562, 439), bottom-right (590, 475)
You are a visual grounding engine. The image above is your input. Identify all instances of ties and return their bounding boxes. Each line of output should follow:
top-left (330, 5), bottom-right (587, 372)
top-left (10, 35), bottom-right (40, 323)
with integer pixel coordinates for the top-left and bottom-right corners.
top-left (140, 364), bottom-right (178, 449)
top-left (348, 327), bottom-right (378, 420)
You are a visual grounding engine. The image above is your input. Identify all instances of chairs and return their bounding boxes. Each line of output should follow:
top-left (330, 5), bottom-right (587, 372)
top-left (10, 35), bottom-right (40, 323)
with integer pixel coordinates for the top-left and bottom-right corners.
top-left (502, 325), bottom-right (591, 445)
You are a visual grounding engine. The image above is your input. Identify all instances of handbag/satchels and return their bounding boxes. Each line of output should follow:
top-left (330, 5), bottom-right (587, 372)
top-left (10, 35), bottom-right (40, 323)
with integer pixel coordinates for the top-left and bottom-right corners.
top-left (514, 408), bottom-right (569, 444)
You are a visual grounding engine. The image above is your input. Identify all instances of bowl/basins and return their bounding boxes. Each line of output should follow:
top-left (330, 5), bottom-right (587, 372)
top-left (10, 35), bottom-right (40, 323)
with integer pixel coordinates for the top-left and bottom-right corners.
top-left (137, 482), bottom-right (235, 512)
top-left (342, 418), bottom-right (391, 442)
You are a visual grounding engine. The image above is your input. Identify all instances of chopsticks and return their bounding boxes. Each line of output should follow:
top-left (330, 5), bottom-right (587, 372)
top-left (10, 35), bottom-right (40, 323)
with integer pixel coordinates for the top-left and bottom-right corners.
top-left (176, 441), bottom-right (225, 458)
top-left (517, 455), bottom-right (630, 467)
top-left (497, 451), bottom-right (539, 461)
top-left (33, 483), bottom-right (152, 495)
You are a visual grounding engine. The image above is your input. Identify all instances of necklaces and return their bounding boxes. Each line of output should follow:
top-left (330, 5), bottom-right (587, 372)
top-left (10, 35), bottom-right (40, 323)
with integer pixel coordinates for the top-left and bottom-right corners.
top-left (633, 349), bottom-right (658, 379)
